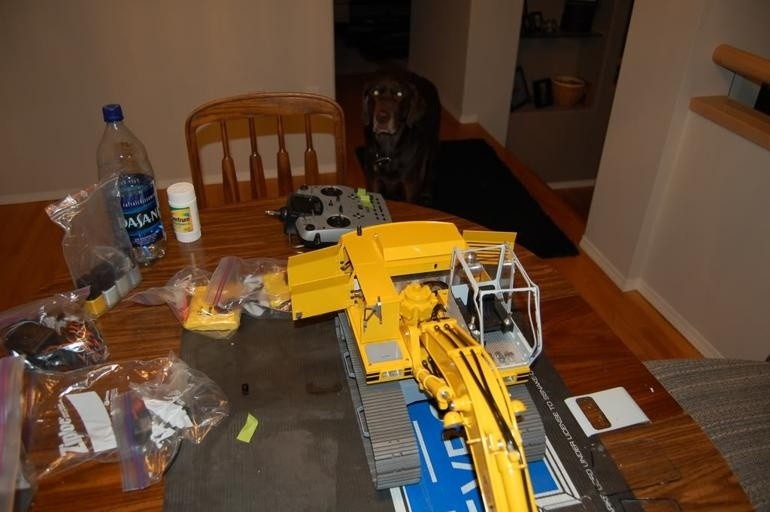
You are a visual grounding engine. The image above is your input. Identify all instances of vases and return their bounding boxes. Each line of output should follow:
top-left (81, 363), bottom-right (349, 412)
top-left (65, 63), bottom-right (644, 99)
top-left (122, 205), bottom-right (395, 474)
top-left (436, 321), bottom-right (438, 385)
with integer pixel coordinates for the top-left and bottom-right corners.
top-left (552, 76), bottom-right (585, 107)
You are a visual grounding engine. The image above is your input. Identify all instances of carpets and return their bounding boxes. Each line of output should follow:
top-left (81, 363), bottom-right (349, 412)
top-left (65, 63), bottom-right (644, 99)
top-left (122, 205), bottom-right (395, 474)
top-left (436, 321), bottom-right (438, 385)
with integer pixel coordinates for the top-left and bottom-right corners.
top-left (356, 136), bottom-right (579, 259)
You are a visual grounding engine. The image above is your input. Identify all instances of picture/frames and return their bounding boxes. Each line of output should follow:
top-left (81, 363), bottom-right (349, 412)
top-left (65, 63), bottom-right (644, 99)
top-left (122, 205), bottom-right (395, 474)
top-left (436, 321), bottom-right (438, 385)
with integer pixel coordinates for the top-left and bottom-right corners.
top-left (528, 12), bottom-right (543, 31)
top-left (510, 65), bottom-right (531, 111)
top-left (531, 77), bottom-right (554, 109)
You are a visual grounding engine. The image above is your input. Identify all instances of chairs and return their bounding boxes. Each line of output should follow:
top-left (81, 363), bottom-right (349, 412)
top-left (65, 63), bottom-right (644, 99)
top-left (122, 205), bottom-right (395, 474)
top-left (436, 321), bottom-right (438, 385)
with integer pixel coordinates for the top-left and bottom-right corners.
top-left (642, 354), bottom-right (769, 511)
top-left (184, 94), bottom-right (347, 210)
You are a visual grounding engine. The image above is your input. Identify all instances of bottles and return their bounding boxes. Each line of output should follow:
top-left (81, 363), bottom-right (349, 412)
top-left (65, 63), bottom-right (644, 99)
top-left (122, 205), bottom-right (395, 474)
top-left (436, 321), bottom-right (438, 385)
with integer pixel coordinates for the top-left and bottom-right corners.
top-left (166, 180), bottom-right (202, 244)
top-left (95, 101), bottom-right (169, 272)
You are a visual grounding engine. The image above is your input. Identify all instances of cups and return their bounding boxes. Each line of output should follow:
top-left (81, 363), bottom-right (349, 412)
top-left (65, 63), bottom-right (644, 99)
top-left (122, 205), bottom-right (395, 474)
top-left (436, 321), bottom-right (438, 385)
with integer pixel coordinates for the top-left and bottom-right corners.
top-left (548, 75), bottom-right (590, 108)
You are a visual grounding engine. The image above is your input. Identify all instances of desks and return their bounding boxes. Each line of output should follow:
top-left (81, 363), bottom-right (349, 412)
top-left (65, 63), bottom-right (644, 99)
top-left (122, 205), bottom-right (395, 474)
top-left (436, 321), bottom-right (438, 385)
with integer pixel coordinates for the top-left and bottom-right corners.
top-left (21, 188), bottom-right (758, 512)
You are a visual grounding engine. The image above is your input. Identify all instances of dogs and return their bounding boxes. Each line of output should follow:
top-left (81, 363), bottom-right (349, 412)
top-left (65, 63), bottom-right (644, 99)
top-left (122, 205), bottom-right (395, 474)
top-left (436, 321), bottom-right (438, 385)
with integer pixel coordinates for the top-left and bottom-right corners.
top-left (361, 66), bottom-right (442, 205)
